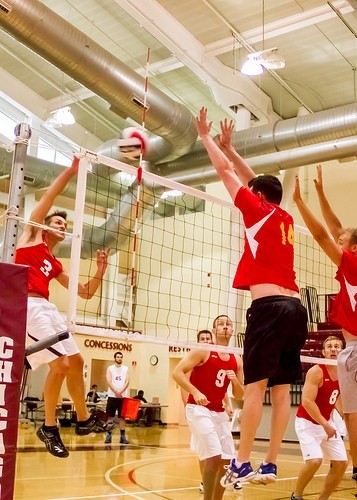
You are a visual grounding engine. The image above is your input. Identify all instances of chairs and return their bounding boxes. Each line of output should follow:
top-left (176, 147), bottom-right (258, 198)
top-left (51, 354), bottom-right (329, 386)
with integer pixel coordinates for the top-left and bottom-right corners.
top-left (24, 397), bottom-right (39, 421)
top-left (61, 399), bottom-right (73, 419)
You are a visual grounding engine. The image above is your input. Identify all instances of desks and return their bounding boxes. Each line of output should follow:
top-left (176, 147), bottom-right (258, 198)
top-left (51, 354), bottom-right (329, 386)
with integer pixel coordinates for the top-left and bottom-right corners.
top-left (137, 402), bottom-right (169, 425)
top-left (20, 399), bottom-right (74, 430)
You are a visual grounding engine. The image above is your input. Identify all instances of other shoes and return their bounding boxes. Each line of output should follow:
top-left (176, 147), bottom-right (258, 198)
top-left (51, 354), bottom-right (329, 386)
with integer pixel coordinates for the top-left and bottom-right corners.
top-left (290, 492), bottom-right (304, 500)
top-left (119, 435), bottom-right (129, 444)
top-left (104, 435), bottom-right (111, 444)
top-left (198, 481), bottom-right (205, 494)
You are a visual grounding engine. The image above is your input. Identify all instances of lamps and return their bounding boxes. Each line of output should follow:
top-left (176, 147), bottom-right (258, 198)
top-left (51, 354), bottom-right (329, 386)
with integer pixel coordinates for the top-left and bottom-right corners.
top-left (43, 71), bottom-right (75, 127)
top-left (239, 0), bottom-right (286, 76)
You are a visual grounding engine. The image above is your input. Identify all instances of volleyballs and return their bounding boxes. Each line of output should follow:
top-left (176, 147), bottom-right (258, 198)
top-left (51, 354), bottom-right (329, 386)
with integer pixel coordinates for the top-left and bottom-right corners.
top-left (118, 127), bottom-right (147, 160)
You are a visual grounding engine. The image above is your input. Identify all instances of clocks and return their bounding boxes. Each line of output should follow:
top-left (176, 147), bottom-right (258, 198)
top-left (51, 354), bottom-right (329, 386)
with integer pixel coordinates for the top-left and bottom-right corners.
top-left (150, 355), bottom-right (158, 365)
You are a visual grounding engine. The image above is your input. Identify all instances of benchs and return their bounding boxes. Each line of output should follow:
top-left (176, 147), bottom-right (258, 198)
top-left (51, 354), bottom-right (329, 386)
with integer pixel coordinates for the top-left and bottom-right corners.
top-left (297, 322), bottom-right (346, 381)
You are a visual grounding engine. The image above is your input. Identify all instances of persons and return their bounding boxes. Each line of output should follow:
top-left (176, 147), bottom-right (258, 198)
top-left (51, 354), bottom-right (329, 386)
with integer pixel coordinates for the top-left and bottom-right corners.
top-left (87, 384), bottom-right (104, 419)
top-left (293, 164), bottom-right (357, 480)
top-left (197, 329), bottom-right (242, 492)
top-left (172, 315), bottom-right (244, 500)
top-left (133, 390), bottom-right (147, 426)
top-left (196, 106), bottom-right (307, 488)
top-left (14, 157), bottom-right (116, 458)
top-left (105, 352), bottom-right (129, 444)
top-left (291, 336), bottom-right (348, 500)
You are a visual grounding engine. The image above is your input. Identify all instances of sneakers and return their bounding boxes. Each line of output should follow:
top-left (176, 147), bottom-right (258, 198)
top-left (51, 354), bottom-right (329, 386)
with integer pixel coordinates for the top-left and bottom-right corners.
top-left (248, 460), bottom-right (278, 484)
top-left (74, 414), bottom-right (115, 436)
top-left (220, 458), bottom-right (256, 488)
top-left (36, 423), bottom-right (69, 458)
